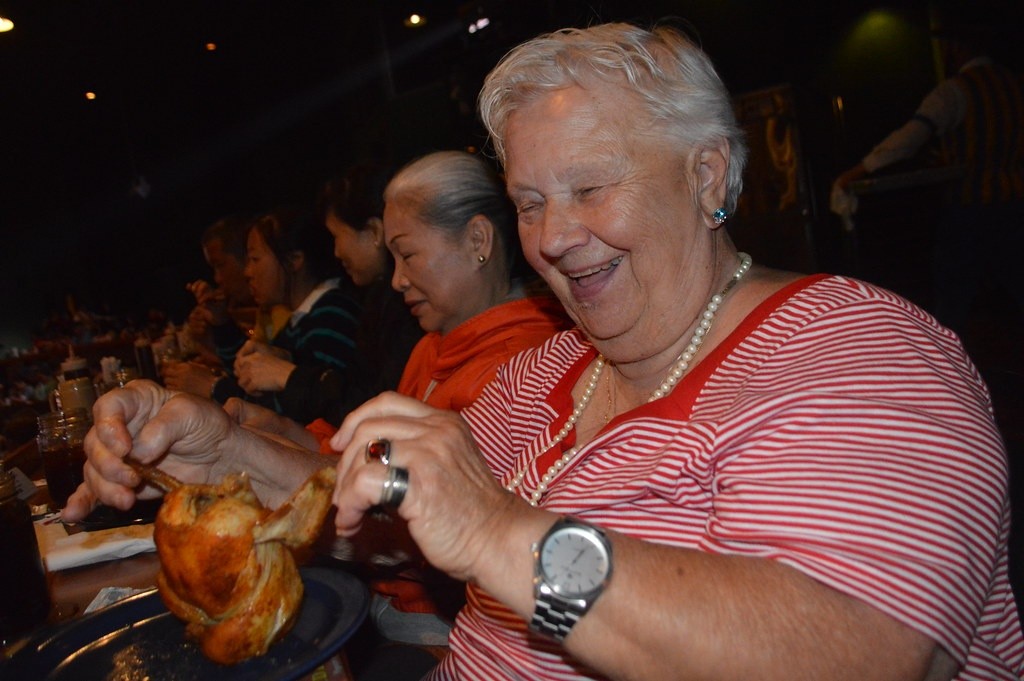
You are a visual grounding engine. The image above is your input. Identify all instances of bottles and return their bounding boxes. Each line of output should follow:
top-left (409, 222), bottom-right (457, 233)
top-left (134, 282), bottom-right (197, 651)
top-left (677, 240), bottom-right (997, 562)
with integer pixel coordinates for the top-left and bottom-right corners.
top-left (59, 342), bottom-right (93, 380)
top-left (35, 409), bottom-right (91, 502)
top-left (135, 331), bottom-right (157, 380)
top-left (0, 459), bottom-right (53, 637)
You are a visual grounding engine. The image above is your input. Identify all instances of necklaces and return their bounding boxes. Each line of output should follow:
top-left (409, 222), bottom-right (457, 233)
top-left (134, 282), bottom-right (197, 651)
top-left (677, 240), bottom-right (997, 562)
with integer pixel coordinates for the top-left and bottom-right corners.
top-left (504, 251), bottom-right (752, 508)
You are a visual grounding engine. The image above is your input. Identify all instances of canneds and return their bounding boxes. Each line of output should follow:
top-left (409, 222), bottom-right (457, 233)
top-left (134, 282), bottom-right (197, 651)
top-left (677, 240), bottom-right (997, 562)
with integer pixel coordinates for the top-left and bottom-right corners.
top-left (36, 405), bottom-right (95, 507)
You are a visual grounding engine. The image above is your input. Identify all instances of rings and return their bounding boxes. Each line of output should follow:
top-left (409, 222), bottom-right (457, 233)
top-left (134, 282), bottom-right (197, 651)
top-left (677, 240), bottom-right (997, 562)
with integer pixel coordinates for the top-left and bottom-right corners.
top-left (379, 466), bottom-right (409, 510)
top-left (365, 438), bottom-right (392, 465)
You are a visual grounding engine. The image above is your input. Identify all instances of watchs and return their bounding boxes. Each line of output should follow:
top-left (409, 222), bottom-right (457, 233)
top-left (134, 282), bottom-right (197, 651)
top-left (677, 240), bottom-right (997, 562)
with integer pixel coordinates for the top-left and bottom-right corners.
top-left (527, 513), bottom-right (615, 645)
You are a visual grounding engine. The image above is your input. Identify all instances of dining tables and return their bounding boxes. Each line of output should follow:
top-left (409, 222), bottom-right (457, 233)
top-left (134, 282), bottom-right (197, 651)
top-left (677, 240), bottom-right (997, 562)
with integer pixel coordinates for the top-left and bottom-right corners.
top-left (0, 438), bottom-right (352, 680)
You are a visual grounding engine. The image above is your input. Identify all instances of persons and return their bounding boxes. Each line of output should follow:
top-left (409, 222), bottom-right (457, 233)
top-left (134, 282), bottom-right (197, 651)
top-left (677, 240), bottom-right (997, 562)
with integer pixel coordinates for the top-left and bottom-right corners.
top-left (60, 0), bottom-right (1024, 681)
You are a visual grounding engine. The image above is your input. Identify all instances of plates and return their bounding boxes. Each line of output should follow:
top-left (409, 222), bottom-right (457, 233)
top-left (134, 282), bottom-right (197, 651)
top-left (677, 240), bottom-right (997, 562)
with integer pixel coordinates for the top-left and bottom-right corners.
top-left (80, 500), bottom-right (159, 528)
top-left (0, 561), bottom-right (375, 681)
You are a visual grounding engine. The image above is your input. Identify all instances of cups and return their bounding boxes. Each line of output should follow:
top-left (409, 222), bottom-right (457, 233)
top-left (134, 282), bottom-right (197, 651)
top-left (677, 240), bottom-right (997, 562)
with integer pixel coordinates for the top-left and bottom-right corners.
top-left (49, 377), bottom-right (99, 421)
